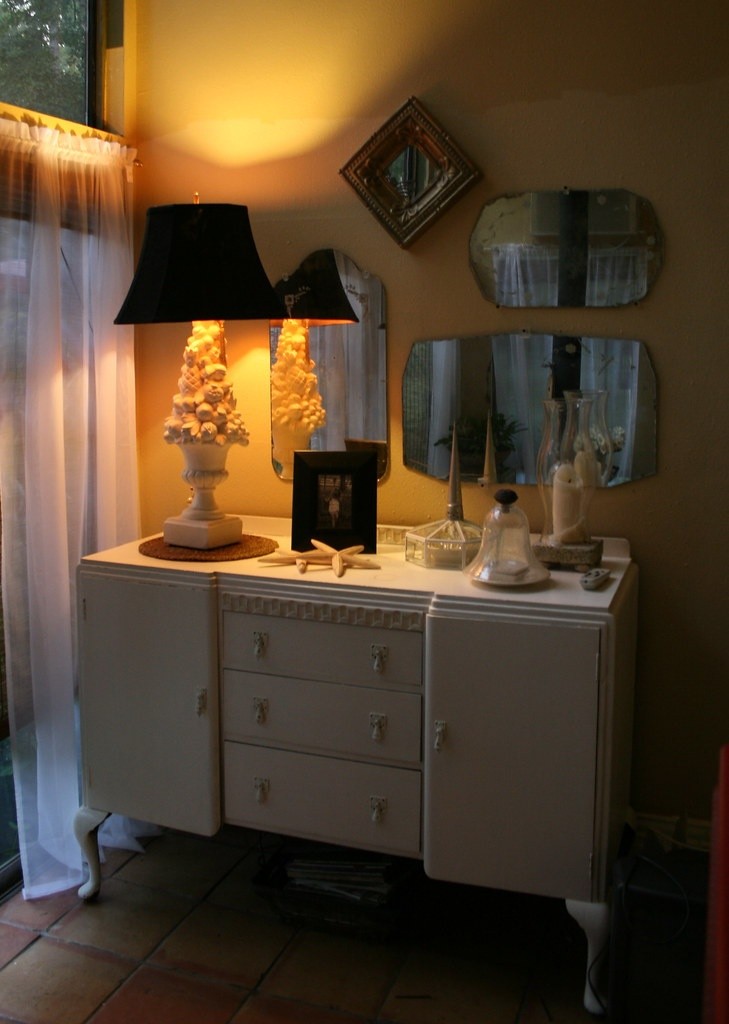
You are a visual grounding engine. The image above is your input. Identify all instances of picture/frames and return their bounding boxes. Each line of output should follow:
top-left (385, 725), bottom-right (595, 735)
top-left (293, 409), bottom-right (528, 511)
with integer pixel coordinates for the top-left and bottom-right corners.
top-left (290, 451), bottom-right (378, 554)
top-left (344, 438), bottom-right (387, 479)
top-left (337, 95), bottom-right (483, 250)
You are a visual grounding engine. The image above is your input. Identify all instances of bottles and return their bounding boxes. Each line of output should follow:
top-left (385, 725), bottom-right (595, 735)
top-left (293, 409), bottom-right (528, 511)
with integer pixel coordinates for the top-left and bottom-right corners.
top-left (534, 397), bottom-right (600, 546)
top-left (563, 389), bottom-right (615, 486)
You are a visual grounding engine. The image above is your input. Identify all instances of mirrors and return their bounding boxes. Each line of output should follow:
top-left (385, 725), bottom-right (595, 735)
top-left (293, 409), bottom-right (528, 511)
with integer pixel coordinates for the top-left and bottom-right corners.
top-left (270, 248), bottom-right (387, 485)
top-left (468, 187), bottom-right (666, 310)
top-left (402, 332), bottom-right (658, 489)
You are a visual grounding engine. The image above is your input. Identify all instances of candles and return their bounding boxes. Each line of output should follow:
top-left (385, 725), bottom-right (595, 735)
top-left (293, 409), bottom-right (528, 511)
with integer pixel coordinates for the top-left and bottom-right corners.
top-left (551, 466), bottom-right (581, 535)
top-left (575, 448), bottom-right (599, 484)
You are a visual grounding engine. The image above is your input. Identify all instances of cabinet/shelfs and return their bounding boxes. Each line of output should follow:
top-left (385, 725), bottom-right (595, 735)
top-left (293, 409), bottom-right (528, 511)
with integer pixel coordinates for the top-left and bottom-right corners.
top-left (71, 513), bottom-right (633, 1014)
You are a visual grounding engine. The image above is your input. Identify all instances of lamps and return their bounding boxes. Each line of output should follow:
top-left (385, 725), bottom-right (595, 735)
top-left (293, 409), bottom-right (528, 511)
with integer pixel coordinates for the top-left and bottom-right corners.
top-left (114, 193), bottom-right (281, 550)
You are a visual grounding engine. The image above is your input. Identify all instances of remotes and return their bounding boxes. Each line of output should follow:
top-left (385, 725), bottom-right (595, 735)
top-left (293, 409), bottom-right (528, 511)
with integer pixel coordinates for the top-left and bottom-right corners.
top-left (580, 568), bottom-right (611, 590)
